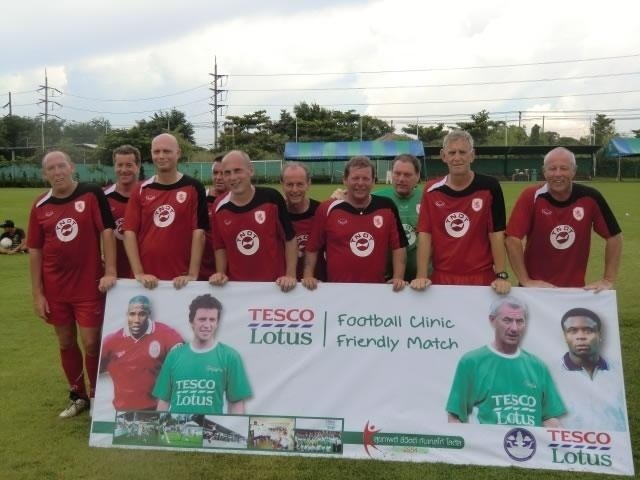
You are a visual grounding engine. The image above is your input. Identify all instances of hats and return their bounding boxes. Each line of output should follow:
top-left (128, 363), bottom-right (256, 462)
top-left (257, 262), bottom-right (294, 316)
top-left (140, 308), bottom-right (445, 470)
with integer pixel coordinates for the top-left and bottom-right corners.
top-left (0, 221), bottom-right (14, 227)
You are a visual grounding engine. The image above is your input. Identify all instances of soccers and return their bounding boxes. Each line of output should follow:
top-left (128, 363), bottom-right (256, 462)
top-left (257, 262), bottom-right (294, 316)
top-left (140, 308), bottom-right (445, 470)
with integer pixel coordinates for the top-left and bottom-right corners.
top-left (1, 238), bottom-right (13, 250)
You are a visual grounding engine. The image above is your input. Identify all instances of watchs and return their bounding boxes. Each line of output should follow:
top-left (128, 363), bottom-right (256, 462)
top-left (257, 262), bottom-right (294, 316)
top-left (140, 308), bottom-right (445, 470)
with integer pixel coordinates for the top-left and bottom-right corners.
top-left (497, 272), bottom-right (509, 280)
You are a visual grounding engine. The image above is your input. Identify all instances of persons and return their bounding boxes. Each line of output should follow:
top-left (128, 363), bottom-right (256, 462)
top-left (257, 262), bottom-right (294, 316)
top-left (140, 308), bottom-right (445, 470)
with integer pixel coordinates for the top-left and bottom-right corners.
top-left (280, 161), bottom-right (325, 283)
top-left (160, 423), bottom-right (169, 443)
top-left (197, 156), bottom-right (227, 281)
top-left (445, 295), bottom-right (567, 429)
top-left (99, 295), bottom-right (186, 417)
top-left (0, 220), bottom-right (30, 255)
top-left (149, 294), bottom-right (254, 414)
top-left (99, 144), bottom-right (143, 279)
top-left (128, 420), bottom-right (152, 441)
top-left (548, 308), bottom-right (626, 431)
top-left (207, 423), bottom-right (218, 444)
top-left (410, 129), bottom-right (512, 294)
top-left (122, 132), bottom-right (209, 289)
top-left (295, 431), bottom-right (341, 453)
top-left (330, 154), bottom-right (433, 283)
top-left (301, 155), bottom-right (410, 293)
top-left (504, 147), bottom-right (623, 294)
top-left (26, 151), bottom-right (117, 417)
top-left (251, 421), bottom-right (289, 450)
top-left (208, 150), bottom-right (297, 293)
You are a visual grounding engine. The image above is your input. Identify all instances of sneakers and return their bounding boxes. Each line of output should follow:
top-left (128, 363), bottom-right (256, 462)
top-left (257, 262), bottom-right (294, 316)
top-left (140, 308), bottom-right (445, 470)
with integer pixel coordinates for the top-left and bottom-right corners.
top-left (59, 392), bottom-right (90, 419)
